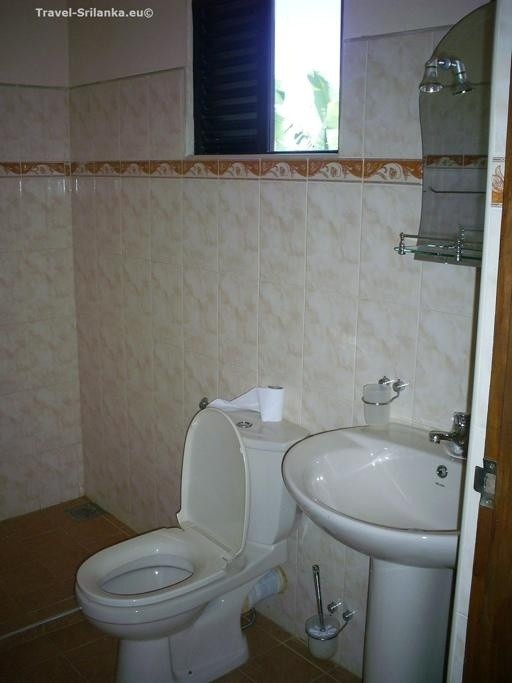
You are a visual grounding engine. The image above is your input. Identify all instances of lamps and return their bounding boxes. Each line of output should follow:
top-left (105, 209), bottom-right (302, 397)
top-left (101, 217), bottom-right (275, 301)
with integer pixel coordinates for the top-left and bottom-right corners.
top-left (451, 57), bottom-right (475, 97)
top-left (419, 57), bottom-right (451, 94)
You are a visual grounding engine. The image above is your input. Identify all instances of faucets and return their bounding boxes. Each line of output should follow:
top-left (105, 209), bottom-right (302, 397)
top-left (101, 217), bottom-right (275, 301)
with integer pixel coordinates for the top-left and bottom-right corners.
top-left (428, 413), bottom-right (470, 455)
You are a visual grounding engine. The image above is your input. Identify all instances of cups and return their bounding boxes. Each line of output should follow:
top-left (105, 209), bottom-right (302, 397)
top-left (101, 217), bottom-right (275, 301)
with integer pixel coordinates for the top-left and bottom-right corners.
top-left (361, 380), bottom-right (393, 432)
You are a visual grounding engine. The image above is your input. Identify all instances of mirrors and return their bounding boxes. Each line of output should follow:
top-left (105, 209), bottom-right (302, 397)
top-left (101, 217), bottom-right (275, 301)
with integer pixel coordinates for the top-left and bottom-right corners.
top-left (414, 1), bottom-right (496, 267)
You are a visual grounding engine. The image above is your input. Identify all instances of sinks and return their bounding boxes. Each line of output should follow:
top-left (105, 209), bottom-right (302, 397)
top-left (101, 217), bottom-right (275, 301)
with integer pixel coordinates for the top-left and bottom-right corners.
top-left (282, 423), bottom-right (465, 537)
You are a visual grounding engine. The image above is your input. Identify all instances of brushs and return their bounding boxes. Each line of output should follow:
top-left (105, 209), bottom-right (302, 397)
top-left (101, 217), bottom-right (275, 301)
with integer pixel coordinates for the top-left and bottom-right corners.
top-left (308, 564), bottom-right (337, 636)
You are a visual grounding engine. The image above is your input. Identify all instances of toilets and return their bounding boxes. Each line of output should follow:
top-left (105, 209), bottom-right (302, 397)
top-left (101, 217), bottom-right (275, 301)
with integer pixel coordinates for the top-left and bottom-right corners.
top-left (74, 408), bottom-right (309, 683)
